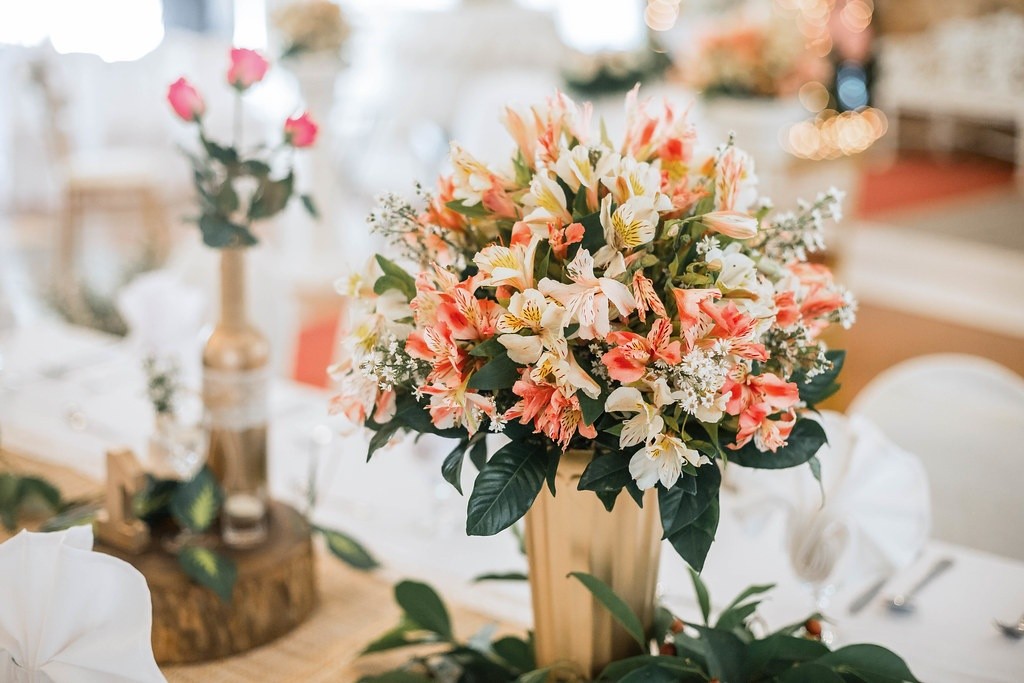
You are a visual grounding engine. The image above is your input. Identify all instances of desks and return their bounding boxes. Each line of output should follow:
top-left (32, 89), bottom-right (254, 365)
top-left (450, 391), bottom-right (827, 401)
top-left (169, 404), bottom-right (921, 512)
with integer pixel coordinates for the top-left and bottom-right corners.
top-left (0, 444), bottom-right (544, 683)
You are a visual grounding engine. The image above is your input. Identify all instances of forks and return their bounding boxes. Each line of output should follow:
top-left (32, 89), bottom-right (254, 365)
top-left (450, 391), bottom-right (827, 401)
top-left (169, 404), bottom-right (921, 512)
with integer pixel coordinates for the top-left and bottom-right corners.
top-left (880, 560), bottom-right (951, 614)
top-left (993, 614), bottom-right (1024, 639)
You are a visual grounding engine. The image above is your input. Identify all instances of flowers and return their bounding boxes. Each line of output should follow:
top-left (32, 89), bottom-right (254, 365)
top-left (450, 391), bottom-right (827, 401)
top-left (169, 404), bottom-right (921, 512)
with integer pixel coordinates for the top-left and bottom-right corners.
top-left (169, 46), bottom-right (325, 249)
top-left (330, 82), bottom-right (860, 573)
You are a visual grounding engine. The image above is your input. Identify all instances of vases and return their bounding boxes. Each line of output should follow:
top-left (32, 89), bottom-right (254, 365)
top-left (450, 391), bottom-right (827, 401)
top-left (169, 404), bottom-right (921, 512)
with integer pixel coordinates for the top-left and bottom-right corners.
top-left (202, 233), bottom-right (272, 500)
top-left (526, 448), bottom-right (665, 683)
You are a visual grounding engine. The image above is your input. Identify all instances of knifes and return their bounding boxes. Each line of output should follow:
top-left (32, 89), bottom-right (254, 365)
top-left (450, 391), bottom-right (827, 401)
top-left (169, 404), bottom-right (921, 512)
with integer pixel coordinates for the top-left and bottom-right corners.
top-left (849, 571), bottom-right (885, 616)
